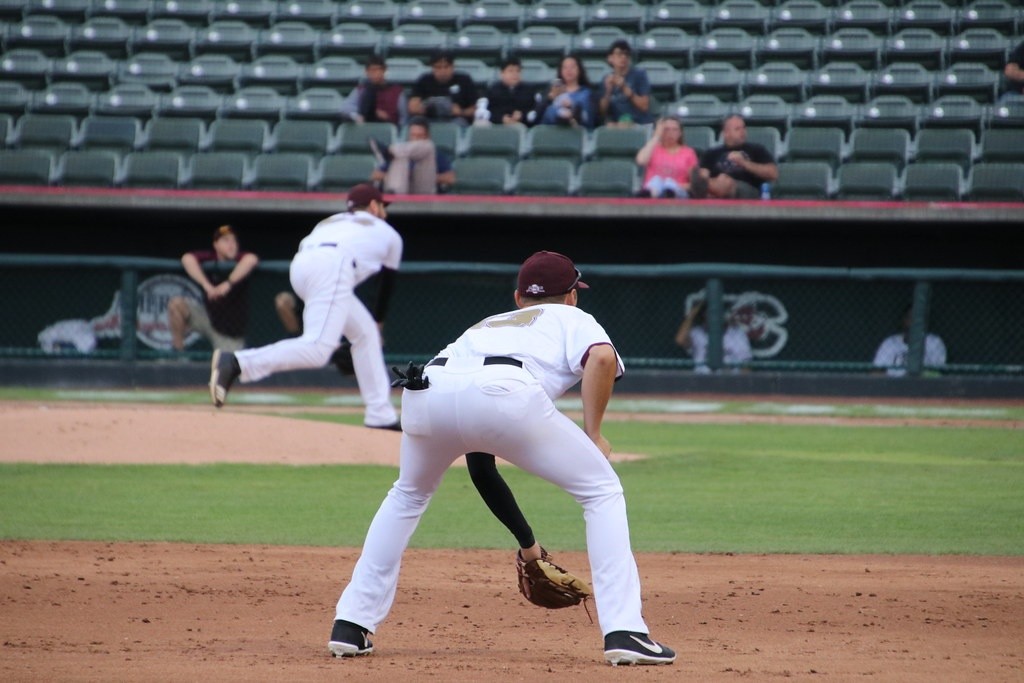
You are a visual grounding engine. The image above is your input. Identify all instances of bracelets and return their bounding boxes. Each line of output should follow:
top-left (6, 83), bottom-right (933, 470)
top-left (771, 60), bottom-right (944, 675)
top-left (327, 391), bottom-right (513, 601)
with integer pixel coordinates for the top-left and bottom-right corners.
top-left (226, 279), bottom-right (234, 288)
top-left (619, 82), bottom-right (626, 89)
top-left (628, 89), bottom-right (636, 101)
top-left (460, 106), bottom-right (465, 118)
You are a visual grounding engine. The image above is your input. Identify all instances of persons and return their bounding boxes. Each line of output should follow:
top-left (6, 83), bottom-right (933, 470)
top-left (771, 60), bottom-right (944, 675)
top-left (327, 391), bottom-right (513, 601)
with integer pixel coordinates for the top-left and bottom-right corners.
top-left (344, 39), bottom-right (651, 133)
top-left (208, 184), bottom-right (404, 432)
top-left (329, 249), bottom-right (677, 667)
top-left (678, 299), bottom-right (754, 377)
top-left (1004, 40), bottom-right (1024, 94)
top-left (368, 117), bottom-right (454, 207)
top-left (169, 224), bottom-right (260, 353)
top-left (635, 112), bottom-right (779, 198)
top-left (874, 305), bottom-right (946, 378)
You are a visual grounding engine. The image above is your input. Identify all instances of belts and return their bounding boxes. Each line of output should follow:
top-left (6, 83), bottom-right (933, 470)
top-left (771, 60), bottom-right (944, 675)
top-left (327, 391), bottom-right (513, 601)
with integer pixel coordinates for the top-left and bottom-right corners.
top-left (426, 356), bottom-right (522, 370)
top-left (319, 241), bottom-right (357, 269)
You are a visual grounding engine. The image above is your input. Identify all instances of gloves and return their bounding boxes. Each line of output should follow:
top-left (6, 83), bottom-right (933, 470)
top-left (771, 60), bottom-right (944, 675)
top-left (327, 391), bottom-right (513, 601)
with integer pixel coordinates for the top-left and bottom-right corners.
top-left (390, 361), bottom-right (428, 390)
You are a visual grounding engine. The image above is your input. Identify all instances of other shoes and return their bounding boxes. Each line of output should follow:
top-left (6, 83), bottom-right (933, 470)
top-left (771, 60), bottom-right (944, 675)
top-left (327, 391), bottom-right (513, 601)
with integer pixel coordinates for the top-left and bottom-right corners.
top-left (369, 136), bottom-right (391, 171)
top-left (689, 167), bottom-right (708, 198)
top-left (659, 189), bottom-right (674, 198)
top-left (635, 189), bottom-right (650, 197)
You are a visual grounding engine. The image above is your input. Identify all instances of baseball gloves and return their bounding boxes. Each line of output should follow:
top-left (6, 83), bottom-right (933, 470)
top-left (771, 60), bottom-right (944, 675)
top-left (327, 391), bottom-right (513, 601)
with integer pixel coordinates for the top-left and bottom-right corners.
top-left (515, 546), bottom-right (594, 612)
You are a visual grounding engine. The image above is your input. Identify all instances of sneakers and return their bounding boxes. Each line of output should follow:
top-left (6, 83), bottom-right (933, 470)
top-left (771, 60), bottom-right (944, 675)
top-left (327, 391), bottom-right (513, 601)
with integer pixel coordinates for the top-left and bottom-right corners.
top-left (365, 421), bottom-right (403, 431)
top-left (604, 631), bottom-right (677, 667)
top-left (210, 348), bottom-right (241, 408)
top-left (328, 620), bottom-right (374, 658)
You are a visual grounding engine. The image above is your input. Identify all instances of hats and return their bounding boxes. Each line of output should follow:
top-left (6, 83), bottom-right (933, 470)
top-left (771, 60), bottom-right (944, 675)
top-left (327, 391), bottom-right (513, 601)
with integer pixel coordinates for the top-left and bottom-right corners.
top-left (346, 183), bottom-right (392, 211)
top-left (214, 225), bottom-right (234, 241)
top-left (517, 251), bottom-right (590, 297)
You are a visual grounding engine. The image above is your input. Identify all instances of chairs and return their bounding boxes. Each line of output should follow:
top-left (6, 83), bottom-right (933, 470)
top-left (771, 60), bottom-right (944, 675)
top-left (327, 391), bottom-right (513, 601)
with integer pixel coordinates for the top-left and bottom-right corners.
top-left (0, 0), bottom-right (1024, 197)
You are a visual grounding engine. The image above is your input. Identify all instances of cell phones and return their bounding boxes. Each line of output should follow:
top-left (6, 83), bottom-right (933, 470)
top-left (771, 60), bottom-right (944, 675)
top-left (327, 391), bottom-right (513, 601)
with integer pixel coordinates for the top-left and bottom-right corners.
top-left (553, 79), bottom-right (561, 88)
